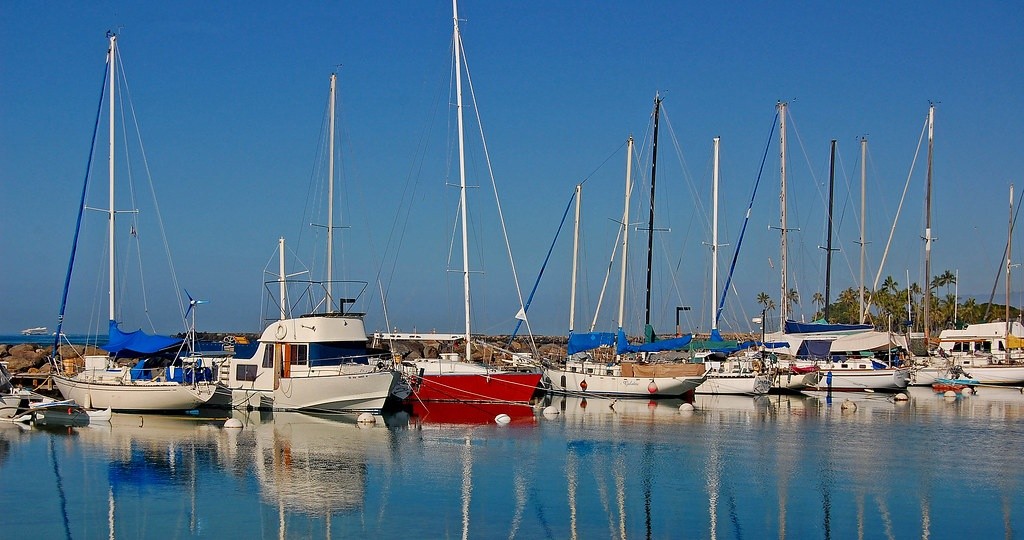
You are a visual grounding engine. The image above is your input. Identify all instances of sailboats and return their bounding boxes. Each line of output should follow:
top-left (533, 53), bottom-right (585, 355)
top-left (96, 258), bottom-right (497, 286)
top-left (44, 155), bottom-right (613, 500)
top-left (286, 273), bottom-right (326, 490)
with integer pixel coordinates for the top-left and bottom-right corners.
top-left (1, 0), bottom-right (1023, 429)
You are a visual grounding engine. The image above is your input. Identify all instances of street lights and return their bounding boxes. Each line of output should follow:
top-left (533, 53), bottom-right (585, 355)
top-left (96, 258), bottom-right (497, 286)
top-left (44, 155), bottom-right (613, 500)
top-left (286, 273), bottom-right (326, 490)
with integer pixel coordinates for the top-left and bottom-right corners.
top-left (338, 298), bottom-right (356, 316)
top-left (675, 305), bottom-right (691, 337)
